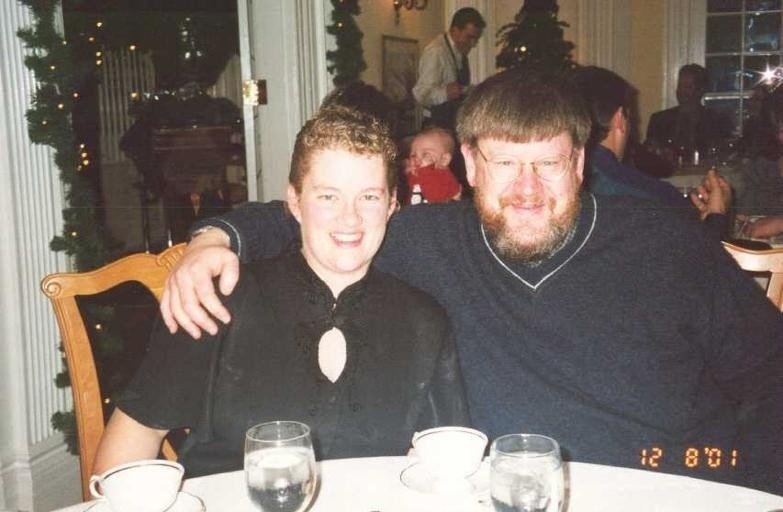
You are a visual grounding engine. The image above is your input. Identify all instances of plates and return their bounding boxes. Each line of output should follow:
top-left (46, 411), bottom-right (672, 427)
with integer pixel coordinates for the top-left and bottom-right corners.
top-left (400, 461), bottom-right (491, 496)
top-left (84, 492), bottom-right (207, 512)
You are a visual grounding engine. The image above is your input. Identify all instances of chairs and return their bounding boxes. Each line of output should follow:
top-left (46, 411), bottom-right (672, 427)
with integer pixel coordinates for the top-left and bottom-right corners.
top-left (38, 241), bottom-right (185, 502)
top-left (721, 240), bottom-right (783, 312)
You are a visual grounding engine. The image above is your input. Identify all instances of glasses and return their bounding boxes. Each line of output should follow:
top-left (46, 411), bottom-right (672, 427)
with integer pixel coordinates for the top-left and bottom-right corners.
top-left (477, 149), bottom-right (574, 180)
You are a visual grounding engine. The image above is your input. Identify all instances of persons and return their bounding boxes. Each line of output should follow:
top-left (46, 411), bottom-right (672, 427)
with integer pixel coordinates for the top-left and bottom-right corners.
top-left (160, 68), bottom-right (783, 495)
top-left (319, 8), bottom-right (783, 244)
top-left (91, 107), bottom-right (472, 495)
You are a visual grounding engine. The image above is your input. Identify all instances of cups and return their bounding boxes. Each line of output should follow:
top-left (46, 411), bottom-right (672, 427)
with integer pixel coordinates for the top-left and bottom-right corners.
top-left (641, 133), bottom-right (741, 171)
top-left (243, 420), bottom-right (319, 512)
top-left (489, 434), bottom-right (566, 512)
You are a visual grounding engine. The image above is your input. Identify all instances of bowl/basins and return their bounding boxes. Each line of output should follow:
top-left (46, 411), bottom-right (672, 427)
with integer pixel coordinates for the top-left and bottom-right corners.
top-left (88, 459), bottom-right (185, 512)
top-left (412, 425), bottom-right (489, 477)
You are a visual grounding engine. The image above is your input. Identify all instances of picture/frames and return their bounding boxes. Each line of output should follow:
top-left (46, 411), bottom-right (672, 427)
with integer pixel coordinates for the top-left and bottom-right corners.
top-left (382, 35), bottom-right (420, 138)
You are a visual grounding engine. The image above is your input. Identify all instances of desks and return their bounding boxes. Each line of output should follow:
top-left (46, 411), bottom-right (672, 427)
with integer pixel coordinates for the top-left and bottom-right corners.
top-left (136, 124), bottom-right (246, 253)
